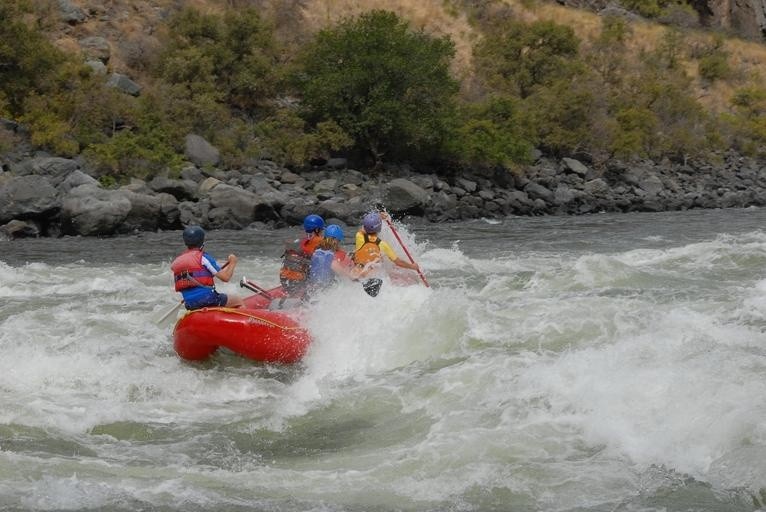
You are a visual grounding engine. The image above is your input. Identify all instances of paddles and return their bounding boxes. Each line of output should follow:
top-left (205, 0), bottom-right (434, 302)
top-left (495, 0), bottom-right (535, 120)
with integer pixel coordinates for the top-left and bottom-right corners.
top-left (160, 262), bottom-right (230, 322)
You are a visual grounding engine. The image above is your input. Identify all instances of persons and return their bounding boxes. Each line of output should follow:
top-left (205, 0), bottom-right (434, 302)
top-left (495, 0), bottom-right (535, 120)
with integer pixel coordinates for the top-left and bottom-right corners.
top-left (302, 212), bottom-right (419, 294)
top-left (170, 226), bottom-right (247, 311)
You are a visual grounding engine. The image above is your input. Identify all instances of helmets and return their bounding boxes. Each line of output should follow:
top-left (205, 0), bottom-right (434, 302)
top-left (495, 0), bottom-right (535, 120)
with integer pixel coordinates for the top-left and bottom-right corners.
top-left (363, 213), bottom-right (383, 234)
top-left (302, 213), bottom-right (325, 234)
top-left (182, 226), bottom-right (206, 248)
top-left (322, 223), bottom-right (345, 241)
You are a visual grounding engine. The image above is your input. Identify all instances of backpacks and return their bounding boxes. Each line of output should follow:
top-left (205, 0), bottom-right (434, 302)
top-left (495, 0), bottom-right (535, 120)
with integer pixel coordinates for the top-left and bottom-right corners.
top-left (279, 238), bottom-right (312, 297)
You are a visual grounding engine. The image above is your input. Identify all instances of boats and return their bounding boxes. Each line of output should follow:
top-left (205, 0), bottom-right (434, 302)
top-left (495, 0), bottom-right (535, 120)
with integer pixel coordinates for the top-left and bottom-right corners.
top-left (171, 258), bottom-right (419, 368)
